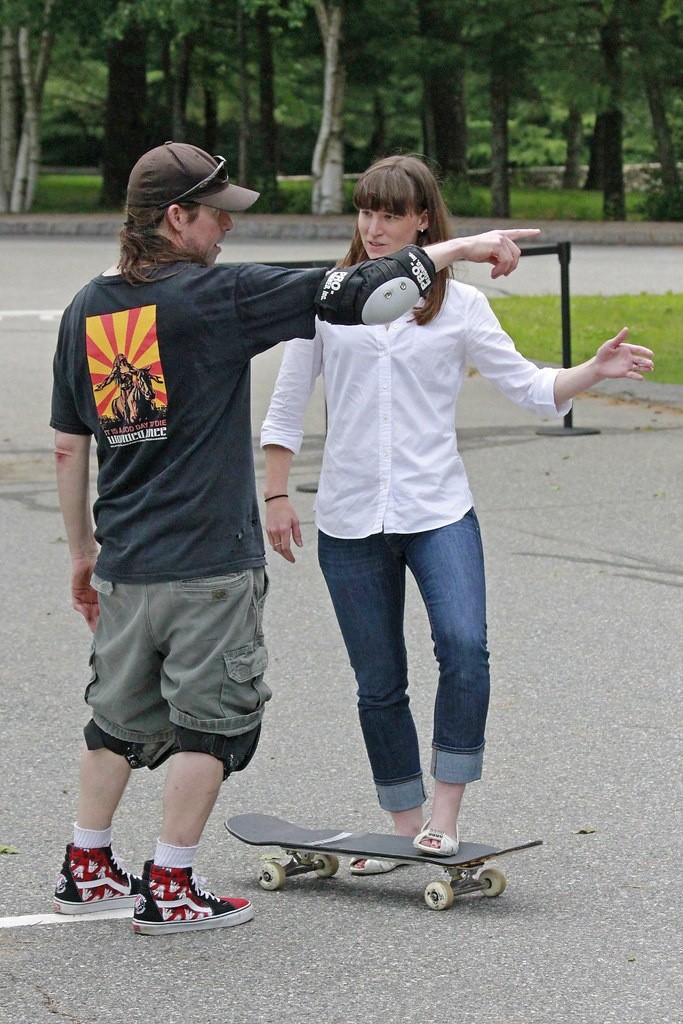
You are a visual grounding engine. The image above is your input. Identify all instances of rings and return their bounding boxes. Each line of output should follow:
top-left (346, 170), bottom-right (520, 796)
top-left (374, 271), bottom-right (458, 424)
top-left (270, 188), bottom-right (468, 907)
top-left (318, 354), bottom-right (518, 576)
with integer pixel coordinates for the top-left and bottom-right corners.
top-left (275, 543), bottom-right (282, 546)
top-left (632, 364), bottom-right (638, 372)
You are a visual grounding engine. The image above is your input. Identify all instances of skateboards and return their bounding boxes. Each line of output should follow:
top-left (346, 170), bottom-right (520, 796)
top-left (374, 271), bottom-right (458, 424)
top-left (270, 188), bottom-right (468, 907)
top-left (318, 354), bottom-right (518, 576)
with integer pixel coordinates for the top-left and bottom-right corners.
top-left (223, 813), bottom-right (546, 910)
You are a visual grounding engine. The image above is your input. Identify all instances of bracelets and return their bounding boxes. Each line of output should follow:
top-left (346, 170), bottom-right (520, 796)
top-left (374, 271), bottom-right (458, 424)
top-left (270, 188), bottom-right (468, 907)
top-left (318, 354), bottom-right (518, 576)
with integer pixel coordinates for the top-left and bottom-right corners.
top-left (264, 495), bottom-right (288, 503)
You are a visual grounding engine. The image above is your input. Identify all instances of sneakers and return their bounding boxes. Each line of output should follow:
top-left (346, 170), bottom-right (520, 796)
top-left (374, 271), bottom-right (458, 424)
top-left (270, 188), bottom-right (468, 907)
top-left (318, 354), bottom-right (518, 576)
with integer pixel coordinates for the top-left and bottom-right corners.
top-left (131, 860), bottom-right (253, 935)
top-left (53, 846), bottom-right (143, 914)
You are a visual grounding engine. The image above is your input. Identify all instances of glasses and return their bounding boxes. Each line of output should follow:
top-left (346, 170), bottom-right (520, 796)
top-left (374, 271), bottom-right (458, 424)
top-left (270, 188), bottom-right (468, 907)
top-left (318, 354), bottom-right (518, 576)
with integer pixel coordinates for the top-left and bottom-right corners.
top-left (157, 155), bottom-right (231, 210)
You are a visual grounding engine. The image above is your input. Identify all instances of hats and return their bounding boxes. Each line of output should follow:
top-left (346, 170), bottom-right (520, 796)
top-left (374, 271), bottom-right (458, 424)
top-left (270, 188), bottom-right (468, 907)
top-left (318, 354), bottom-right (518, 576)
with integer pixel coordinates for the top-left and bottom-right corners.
top-left (124, 140), bottom-right (260, 212)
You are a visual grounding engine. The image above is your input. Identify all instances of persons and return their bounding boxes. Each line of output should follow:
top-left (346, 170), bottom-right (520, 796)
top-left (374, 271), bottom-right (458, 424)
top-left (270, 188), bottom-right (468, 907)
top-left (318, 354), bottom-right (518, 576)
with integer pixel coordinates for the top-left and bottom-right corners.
top-left (48, 142), bottom-right (540, 935)
top-left (264, 156), bottom-right (653, 875)
top-left (265, 494), bottom-right (288, 502)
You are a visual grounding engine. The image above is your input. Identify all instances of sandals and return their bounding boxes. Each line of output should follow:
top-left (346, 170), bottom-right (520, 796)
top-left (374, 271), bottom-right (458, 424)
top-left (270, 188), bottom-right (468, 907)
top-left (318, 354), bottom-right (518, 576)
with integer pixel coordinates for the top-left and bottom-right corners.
top-left (348, 858), bottom-right (402, 875)
top-left (412, 818), bottom-right (460, 856)
